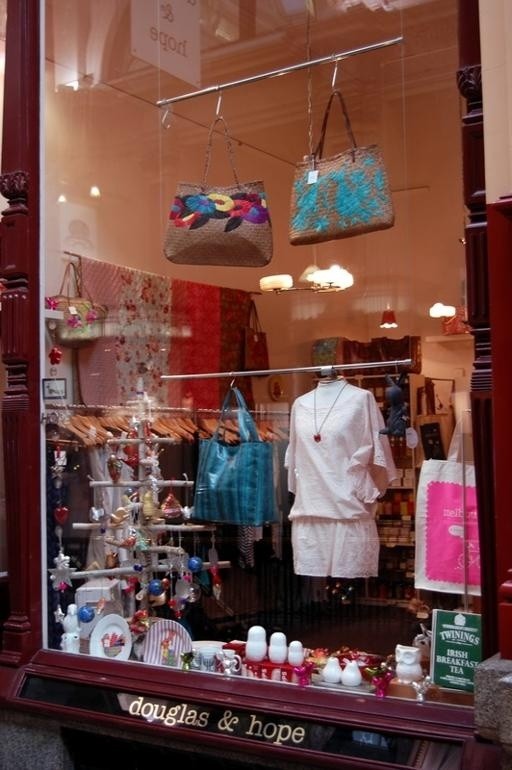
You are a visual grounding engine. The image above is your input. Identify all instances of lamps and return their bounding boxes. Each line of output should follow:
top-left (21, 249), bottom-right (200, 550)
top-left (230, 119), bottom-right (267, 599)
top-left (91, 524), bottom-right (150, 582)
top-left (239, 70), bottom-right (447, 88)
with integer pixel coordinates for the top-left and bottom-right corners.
top-left (378, 303), bottom-right (398, 330)
top-left (258, 154), bottom-right (355, 295)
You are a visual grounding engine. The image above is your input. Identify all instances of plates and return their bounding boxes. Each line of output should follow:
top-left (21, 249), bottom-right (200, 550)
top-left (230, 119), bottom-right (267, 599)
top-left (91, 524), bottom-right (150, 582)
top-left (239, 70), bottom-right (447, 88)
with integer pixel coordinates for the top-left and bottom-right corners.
top-left (141, 619), bottom-right (192, 669)
top-left (88, 614), bottom-right (132, 661)
top-left (270, 374), bottom-right (287, 402)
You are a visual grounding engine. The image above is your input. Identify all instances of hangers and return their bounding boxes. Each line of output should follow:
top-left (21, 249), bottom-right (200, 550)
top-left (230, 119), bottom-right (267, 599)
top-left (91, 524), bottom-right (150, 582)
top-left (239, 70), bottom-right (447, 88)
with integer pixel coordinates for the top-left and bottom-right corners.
top-left (44, 402), bottom-right (290, 450)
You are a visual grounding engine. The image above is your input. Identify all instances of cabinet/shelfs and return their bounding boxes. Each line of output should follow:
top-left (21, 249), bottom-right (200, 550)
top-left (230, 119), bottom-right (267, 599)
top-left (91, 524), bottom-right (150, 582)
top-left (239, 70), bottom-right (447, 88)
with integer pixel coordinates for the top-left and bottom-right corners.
top-left (312, 371), bottom-right (436, 614)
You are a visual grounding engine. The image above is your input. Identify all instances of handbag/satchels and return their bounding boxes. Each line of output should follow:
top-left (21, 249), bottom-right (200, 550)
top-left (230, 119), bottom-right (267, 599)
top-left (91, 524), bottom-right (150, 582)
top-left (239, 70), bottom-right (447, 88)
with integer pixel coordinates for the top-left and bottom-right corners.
top-left (441, 304), bottom-right (470, 335)
top-left (242, 300), bottom-right (270, 377)
top-left (191, 385), bottom-right (279, 528)
top-left (414, 414), bottom-right (482, 596)
top-left (371, 335), bottom-right (423, 374)
top-left (45, 262), bottom-right (108, 349)
top-left (288, 88), bottom-right (396, 246)
top-left (162, 116), bottom-right (274, 267)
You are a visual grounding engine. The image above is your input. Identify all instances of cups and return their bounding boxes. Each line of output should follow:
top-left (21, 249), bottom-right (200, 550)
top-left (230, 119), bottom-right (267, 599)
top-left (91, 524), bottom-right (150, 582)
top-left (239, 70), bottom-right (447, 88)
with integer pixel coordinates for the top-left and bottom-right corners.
top-left (200, 648), bottom-right (242, 675)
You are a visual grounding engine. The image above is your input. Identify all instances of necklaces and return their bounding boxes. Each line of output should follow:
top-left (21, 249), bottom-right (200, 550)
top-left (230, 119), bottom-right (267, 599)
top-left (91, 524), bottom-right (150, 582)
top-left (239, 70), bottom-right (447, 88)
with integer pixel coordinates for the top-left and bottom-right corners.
top-left (312, 382), bottom-right (348, 443)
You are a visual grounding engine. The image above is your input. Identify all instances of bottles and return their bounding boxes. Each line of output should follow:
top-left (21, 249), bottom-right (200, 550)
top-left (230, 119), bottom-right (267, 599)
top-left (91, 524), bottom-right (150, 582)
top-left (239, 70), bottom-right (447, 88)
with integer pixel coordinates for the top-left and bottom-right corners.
top-left (322, 656), bottom-right (362, 688)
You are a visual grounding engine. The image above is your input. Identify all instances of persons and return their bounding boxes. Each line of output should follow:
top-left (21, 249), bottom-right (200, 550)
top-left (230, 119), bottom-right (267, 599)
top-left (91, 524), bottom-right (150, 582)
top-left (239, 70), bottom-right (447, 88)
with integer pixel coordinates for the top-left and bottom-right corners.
top-left (283, 378), bottom-right (396, 580)
